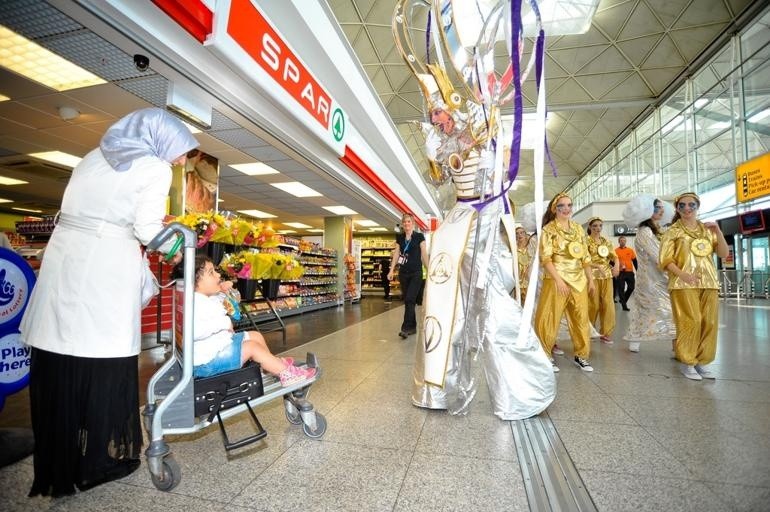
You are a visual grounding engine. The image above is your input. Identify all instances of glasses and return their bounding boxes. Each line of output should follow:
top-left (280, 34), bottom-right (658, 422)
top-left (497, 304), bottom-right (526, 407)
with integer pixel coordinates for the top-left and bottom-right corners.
top-left (677, 202), bottom-right (698, 209)
top-left (591, 223), bottom-right (604, 229)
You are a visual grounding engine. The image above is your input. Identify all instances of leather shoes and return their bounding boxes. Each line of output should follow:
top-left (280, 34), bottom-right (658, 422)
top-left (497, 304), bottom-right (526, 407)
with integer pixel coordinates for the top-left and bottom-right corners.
top-left (398, 328), bottom-right (409, 339)
top-left (74, 454), bottom-right (142, 493)
top-left (408, 328), bottom-right (416, 335)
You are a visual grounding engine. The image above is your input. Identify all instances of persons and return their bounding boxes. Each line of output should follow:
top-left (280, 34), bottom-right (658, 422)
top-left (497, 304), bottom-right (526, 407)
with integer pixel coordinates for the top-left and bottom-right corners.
top-left (536, 191), bottom-right (597, 374)
top-left (623, 190), bottom-right (679, 354)
top-left (15, 106), bottom-right (202, 500)
top-left (194, 252), bottom-right (320, 391)
top-left (386, 212), bottom-right (430, 339)
top-left (584, 217), bottom-right (621, 346)
top-left (410, 65), bottom-right (561, 422)
top-left (512, 222), bottom-right (535, 308)
top-left (657, 192), bottom-right (731, 383)
top-left (612, 234), bottom-right (639, 314)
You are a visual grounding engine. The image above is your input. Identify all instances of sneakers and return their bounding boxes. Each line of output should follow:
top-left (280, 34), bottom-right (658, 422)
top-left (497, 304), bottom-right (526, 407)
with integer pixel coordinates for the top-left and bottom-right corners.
top-left (550, 358), bottom-right (560, 373)
top-left (573, 355), bottom-right (594, 374)
top-left (679, 362), bottom-right (703, 381)
top-left (551, 343), bottom-right (565, 355)
top-left (599, 335), bottom-right (614, 347)
top-left (276, 364), bottom-right (317, 388)
top-left (694, 363), bottom-right (717, 379)
top-left (628, 341), bottom-right (640, 353)
top-left (271, 356), bottom-right (296, 379)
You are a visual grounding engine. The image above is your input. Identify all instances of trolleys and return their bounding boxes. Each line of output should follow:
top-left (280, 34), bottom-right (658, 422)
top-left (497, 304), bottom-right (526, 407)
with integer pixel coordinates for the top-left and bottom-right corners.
top-left (142, 221), bottom-right (326, 494)
